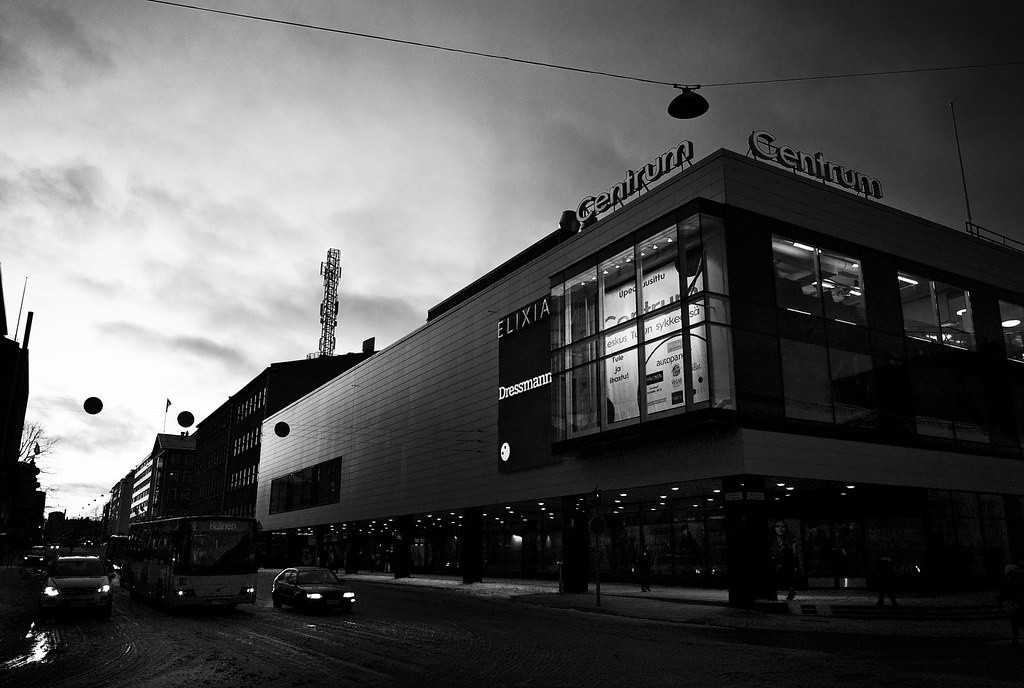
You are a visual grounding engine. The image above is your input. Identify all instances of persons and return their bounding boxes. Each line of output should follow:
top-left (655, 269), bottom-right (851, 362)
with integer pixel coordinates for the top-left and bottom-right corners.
top-left (779, 549), bottom-right (801, 601)
top-left (635, 549), bottom-right (652, 592)
top-left (771, 520), bottom-right (796, 560)
top-left (876, 553), bottom-right (899, 607)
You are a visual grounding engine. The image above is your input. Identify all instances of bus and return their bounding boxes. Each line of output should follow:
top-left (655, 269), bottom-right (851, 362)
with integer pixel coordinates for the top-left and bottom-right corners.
top-left (105, 515), bottom-right (263, 613)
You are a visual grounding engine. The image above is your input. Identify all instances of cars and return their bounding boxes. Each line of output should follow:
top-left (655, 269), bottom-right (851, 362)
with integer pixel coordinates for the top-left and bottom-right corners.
top-left (23, 546), bottom-right (48, 567)
top-left (40, 555), bottom-right (116, 616)
top-left (272, 566), bottom-right (356, 616)
